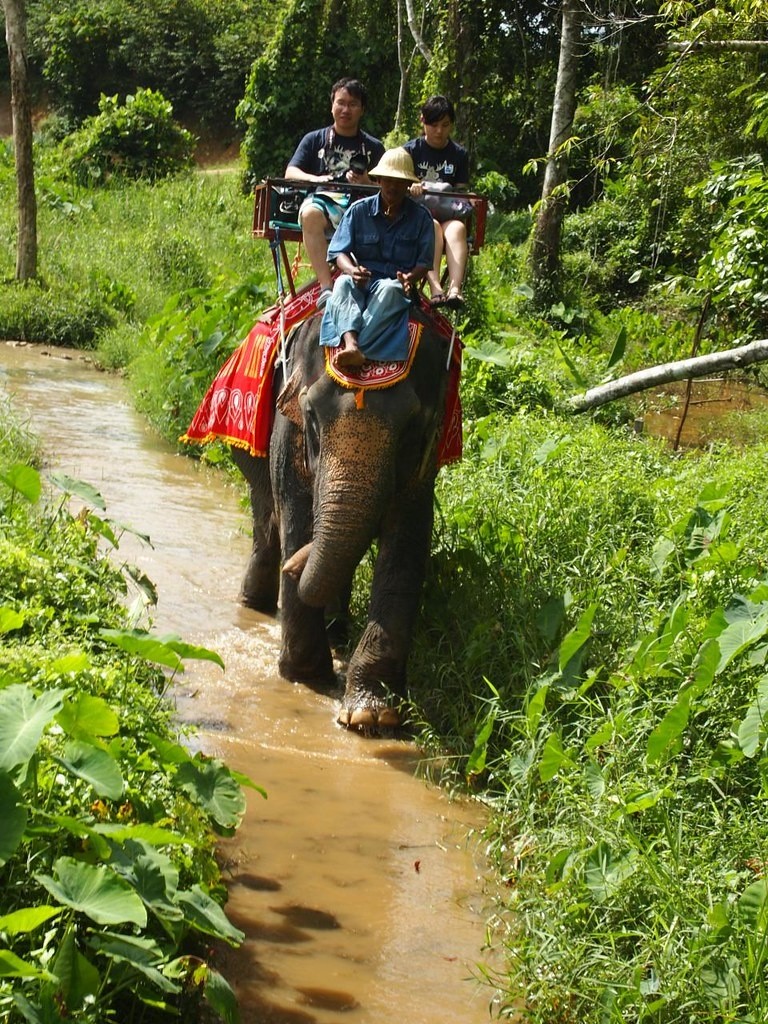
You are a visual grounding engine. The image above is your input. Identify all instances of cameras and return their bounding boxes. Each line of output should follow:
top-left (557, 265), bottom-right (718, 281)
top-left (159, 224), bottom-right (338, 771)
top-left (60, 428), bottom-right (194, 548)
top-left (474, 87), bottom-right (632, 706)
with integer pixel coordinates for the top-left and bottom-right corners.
top-left (333, 154), bottom-right (368, 186)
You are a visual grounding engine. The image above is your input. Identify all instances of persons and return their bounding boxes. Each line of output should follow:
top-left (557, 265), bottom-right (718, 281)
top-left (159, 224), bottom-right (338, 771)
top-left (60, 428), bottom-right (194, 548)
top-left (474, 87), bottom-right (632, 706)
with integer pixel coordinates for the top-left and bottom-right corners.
top-left (284, 80), bottom-right (387, 308)
top-left (325, 145), bottom-right (435, 371)
top-left (400, 96), bottom-right (471, 309)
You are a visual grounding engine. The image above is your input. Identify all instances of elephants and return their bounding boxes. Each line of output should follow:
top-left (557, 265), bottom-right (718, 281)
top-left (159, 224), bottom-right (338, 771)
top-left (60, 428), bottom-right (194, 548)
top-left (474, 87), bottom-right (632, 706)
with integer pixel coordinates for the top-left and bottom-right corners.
top-left (211, 268), bottom-right (464, 741)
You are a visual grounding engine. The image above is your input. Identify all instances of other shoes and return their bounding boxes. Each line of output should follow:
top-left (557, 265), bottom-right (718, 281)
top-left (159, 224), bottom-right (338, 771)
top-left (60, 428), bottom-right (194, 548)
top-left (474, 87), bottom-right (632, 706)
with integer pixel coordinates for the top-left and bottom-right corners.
top-left (315, 289), bottom-right (332, 309)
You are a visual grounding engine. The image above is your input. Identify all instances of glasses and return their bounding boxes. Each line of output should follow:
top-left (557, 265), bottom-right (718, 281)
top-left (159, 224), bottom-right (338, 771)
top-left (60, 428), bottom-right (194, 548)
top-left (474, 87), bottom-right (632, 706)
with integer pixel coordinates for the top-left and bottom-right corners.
top-left (335, 100), bottom-right (361, 110)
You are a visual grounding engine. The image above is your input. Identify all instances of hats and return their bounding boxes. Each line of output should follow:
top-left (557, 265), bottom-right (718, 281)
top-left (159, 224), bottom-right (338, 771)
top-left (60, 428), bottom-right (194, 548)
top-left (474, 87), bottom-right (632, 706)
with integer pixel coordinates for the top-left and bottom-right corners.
top-left (368, 146), bottom-right (421, 184)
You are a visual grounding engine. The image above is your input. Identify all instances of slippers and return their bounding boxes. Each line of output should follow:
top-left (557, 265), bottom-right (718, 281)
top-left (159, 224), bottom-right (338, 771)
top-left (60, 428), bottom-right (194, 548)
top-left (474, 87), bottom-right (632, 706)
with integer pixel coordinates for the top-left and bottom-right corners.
top-left (445, 292), bottom-right (464, 309)
top-left (431, 295), bottom-right (445, 308)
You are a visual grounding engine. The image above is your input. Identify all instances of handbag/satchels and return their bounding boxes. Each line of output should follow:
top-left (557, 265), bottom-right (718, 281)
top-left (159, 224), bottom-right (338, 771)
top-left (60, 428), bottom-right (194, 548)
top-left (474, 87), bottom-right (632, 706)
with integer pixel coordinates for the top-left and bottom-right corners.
top-left (411, 181), bottom-right (472, 219)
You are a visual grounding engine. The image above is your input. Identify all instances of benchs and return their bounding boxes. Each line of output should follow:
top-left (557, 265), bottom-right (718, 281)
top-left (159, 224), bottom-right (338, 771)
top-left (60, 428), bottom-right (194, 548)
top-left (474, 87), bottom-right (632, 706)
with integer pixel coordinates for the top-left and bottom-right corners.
top-left (249, 178), bottom-right (488, 304)
